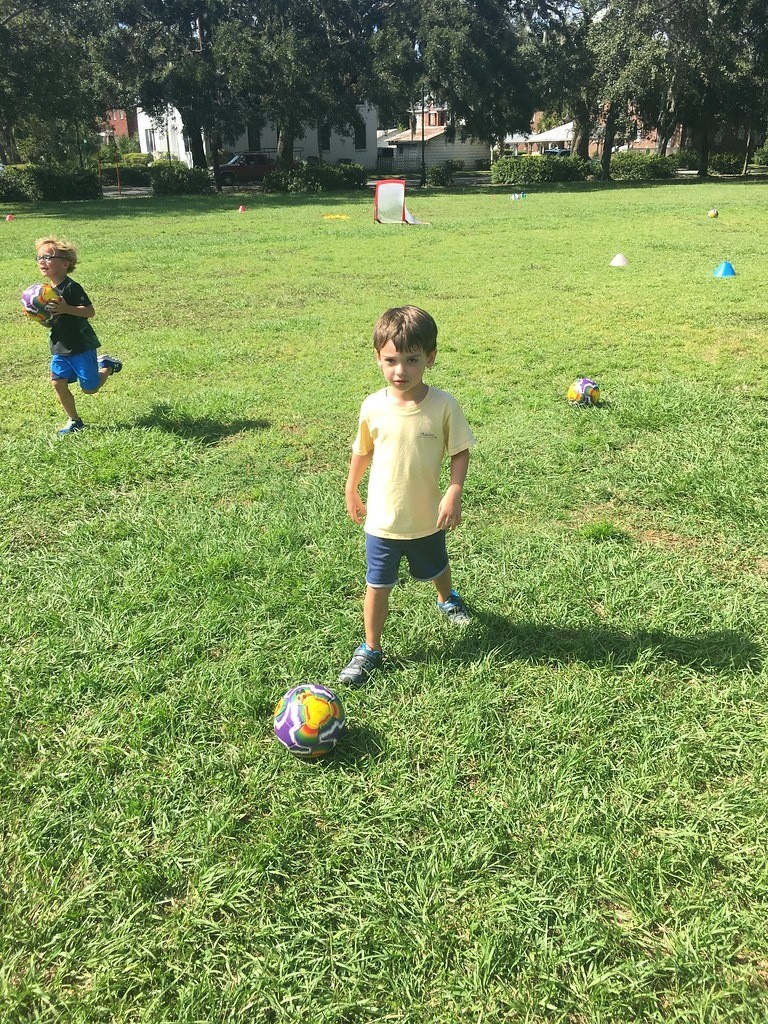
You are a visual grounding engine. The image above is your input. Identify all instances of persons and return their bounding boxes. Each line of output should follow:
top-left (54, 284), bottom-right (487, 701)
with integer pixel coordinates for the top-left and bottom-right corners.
top-left (35, 237), bottom-right (123, 435)
top-left (340, 305), bottom-right (475, 685)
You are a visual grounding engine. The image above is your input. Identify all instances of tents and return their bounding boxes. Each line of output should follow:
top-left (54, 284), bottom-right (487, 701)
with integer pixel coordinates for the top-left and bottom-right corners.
top-left (525, 119), bottom-right (629, 142)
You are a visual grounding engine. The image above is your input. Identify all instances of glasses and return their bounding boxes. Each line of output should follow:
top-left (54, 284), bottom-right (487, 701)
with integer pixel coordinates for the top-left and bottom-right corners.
top-left (36, 255), bottom-right (67, 260)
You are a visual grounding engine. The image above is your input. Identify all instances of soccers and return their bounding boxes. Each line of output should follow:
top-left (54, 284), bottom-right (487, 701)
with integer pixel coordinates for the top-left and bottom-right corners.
top-left (708, 209), bottom-right (719, 218)
top-left (565, 377), bottom-right (600, 408)
top-left (274, 683), bottom-right (345, 760)
top-left (21, 283), bottom-right (59, 322)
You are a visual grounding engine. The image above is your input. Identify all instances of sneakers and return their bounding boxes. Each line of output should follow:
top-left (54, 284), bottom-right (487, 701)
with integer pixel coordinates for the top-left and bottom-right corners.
top-left (97, 354), bottom-right (123, 374)
top-left (436, 589), bottom-right (470, 625)
top-left (58, 417), bottom-right (85, 435)
top-left (337, 642), bottom-right (383, 686)
